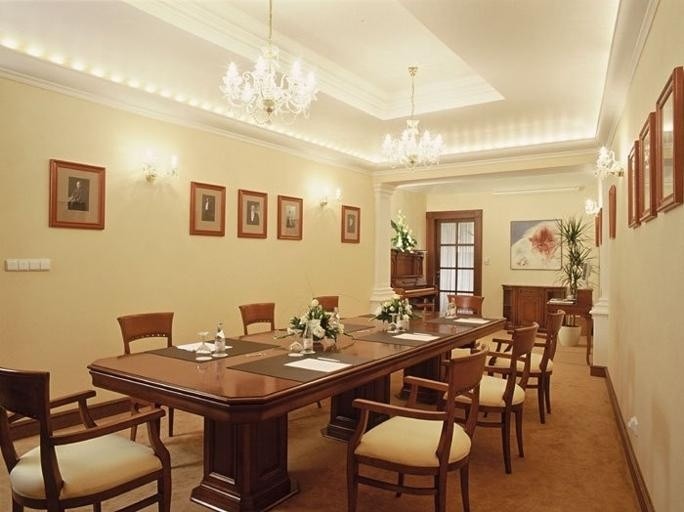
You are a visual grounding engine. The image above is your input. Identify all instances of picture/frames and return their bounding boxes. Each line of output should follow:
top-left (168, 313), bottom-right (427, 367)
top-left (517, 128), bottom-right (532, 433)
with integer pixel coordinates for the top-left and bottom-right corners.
top-left (277, 195), bottom-right (303, 240)
top-left (607, 184), bottom-right (616, 238)
top-left (341, 205), bottom-right (360, 243)
top-left (626, 141), bottom-right (644, 228)
top-left (594, 208), bottom-right (602, 247)
top-left (51, 159), bottom-right (105, 230)
top-left (509, 219), bottom-right (562, 271)
top-left (187, 182), bottom-right (225, 237)
top-left (637, 112), bottom-right (657, 223)
top-left (654, 65), bottom-right (684, 214)
top-left (237, 189), bottom-right (267, 239)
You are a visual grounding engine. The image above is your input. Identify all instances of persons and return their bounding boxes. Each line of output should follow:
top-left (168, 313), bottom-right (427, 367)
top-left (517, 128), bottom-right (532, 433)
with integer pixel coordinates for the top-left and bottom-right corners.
top-left (286, 211), bottom-right (292, 226)
top-left (69, 180), bottom-right (86, 209)
top-left (247, 204), bottom-right (258, 225)
top-left (203, 197), bottom-right (211, 218)
top-left (348, 216), bottom-right (354, 231)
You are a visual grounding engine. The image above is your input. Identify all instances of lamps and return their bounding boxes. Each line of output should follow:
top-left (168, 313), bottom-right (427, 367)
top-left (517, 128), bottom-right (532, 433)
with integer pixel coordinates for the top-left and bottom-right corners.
top-left (319, 185), bottom-right (331, 206)
top-left (380, 66), bottom-right (444, 172)
top-left (590, 146), bottom-right (624, 178)
top-left (218, 0), bottom-right (321, 125)
top-left (142, 147), bottom-right (161, 185)
top-left (585, 197), bottom-right (600, 215)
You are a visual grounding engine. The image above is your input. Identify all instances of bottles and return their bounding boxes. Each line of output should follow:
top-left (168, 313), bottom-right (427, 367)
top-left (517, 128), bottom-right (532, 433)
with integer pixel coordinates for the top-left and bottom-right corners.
top-left (448, 298), bottom-right (456, 317)
top-left (396, 302), bottom-right (406, 331)
top-left (303, 321), bottom-right (313, 353)
top-left (213, 321), bottom-right (227, 356)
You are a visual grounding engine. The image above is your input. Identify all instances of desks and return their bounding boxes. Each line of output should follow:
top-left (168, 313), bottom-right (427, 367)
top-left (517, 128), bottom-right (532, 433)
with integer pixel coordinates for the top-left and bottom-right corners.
top-left (546, 298), bottom-right (591, 365)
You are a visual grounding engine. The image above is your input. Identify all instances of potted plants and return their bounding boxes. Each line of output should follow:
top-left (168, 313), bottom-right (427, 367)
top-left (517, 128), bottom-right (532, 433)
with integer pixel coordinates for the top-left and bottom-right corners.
top-left (553, 215), bottom-right (600, 345)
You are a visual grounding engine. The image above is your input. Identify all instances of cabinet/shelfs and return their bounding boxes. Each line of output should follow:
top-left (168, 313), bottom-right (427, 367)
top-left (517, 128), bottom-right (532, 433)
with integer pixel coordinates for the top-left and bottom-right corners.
top-left (393, 284), bottom-right (438, 311)
top-left (391, 249), bottom-right (424, 289)
top-left (501, 285), bottom-right (593, 332)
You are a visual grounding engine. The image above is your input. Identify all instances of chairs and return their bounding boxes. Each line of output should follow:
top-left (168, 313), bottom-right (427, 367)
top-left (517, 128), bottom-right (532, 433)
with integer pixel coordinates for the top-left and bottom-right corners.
top-left (0, 365), bottom-right (171, 512)
top-left (345, 344), bottom-right (488, 510)
top-left (313, 296), bottom-right (339, 319)
top-left (448, 295), bottom-right (485, 316)
top-left (238, 301), bottom-right (275, 336)
top-left (470, 309), bottom-right (564, 424)
top-left (437, 321), bottom-right (539, 473)
top-left (116, 309), bottom-right (174, 440)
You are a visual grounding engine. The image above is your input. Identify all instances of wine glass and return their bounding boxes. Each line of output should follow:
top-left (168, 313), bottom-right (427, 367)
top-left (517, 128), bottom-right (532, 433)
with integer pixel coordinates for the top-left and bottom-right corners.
top-left (195, 331), bottom-right (213, 362)
top-left (288, 328), bottom-right (303, 354)
top-left (386, 313), bottom-right (399, 334)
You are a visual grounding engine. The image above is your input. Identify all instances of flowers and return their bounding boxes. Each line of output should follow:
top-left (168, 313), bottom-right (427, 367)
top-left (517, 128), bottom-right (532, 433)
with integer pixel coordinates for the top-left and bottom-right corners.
top-left (390, 210), bottom-right (417, 250)
top-left (369, 295), bottom-right (419, 322)
top-left (272, 299), bottom-right (354, 343)
